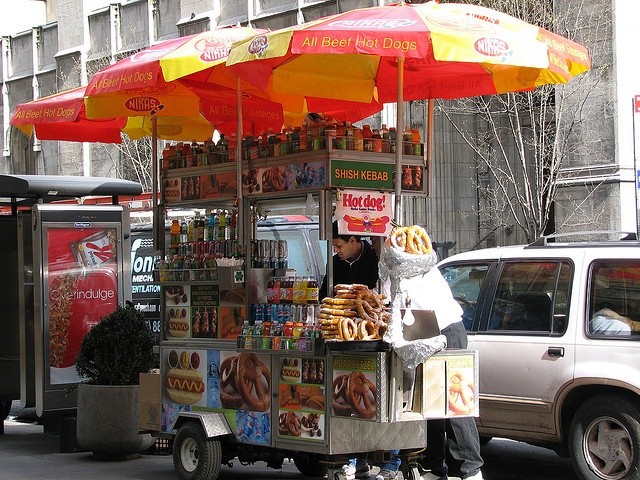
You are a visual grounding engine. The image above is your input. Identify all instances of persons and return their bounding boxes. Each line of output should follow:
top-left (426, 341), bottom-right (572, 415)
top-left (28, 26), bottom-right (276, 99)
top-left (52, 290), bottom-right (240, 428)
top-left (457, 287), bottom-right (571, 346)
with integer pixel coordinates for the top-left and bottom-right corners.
top-left (367, 217), bottom-right (486, 480)
top-left (317, 219), bottom-right (406, 480)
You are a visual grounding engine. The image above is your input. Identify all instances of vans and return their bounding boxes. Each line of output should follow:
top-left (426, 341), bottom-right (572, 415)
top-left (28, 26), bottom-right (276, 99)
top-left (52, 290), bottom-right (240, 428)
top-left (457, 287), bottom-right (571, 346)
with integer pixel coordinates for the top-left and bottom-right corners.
top-left (132, 214), bottom-right (337, 371)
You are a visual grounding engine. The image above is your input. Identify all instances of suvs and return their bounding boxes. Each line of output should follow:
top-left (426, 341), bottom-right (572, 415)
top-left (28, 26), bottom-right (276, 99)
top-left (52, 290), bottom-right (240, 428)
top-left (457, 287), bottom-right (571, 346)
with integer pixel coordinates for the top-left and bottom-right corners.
top-left (435, 230), bottom-right (640, 480)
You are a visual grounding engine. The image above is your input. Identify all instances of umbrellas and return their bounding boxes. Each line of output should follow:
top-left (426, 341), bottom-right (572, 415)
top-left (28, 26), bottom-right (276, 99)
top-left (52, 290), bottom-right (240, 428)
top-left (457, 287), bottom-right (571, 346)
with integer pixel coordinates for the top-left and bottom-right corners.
top-left (81, 19), bottom-right (383, 242)
top-left (225, 0), bottom-right (593, 237)
top-left (11, 84), bottom-right (218, 249)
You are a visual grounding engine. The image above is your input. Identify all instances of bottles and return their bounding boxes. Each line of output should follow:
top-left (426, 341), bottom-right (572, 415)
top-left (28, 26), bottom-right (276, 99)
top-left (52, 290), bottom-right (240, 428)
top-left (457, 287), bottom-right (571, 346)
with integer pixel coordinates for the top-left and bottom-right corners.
top-left (402, 167), bottom-right (412, 189)
top-left (392, 170), bottom-right (395, 189)
top-left (240, 319), bottom-right (321, 352)
top-left (267, 275), bottom-right (319, 304)
top-left (207, 359), bottom-right (221, 409)
top-left (154, 253), bottom-right (218, 282)
top-left (170, 208), bottom-right (239, 246)
top-left (162, 120), bottom-right (422, 171)
top-left (413, 166), bottom-right (421, 189)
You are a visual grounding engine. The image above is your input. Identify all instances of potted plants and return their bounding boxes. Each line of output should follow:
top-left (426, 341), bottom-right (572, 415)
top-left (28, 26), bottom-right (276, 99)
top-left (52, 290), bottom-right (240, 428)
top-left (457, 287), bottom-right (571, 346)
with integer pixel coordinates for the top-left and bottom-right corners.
top-left (75, 306), bottom-right (159, 461)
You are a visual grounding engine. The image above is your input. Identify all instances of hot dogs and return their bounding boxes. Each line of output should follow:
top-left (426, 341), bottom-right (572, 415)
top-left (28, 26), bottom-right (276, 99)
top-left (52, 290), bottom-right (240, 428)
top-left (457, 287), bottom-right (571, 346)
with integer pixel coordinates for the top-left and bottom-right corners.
top-left (164, 187), bottom-right (180, 202)
top-left (283, 367), bottom-right (300, 382)
top-left (168, 318), bottom-right (190, 337)
top-left (166, 369), bottom-right (205, 404)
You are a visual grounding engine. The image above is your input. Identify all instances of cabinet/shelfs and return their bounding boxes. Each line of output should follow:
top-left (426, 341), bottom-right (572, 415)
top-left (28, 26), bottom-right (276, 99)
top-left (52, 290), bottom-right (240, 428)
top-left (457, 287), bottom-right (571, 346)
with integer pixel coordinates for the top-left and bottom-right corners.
top-left (159, 135), bottom-right (429, 352)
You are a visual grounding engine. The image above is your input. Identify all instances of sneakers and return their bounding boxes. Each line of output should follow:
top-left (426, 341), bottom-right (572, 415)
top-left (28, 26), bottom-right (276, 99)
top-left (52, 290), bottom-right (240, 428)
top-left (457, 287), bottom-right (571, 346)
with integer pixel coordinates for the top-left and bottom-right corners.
top-left (376, 468), bottom-right (398, 480)
top-left (356, 465), bottom-right (372, 478)
top-left (419, 472), bottom-right (448, 480)
top-left (463, 471), bottom-right (483, 480)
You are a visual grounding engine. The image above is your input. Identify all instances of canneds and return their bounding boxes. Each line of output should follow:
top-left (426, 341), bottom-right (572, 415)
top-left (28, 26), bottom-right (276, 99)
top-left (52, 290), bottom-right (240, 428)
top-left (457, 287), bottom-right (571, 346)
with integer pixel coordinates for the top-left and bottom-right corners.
top-left (192, 242), bottom-right (199, 254)
top-left (180, 244), bottom-right (183, 255)
top-left (203, 241), bottom-right (208, 250)
top-left (214, 241), bottom-right (218, 253)
top-left (209, 241), bottom-right (214, 251)
top-left (255, 240), bottom-right (262, 256)
top-left (284, 305), bottom-right (290, 323)
top-left (238, 244), bottom-right (246, 255)
top-left (266, 302), bottom-right (271, 321)
top-left (277, 304), bottom-right (284, 323)
top-left (259, 302), bottom-right (266, 320)
top-left (279, 239), bottom-right (288, 258)
top-left (254, 303), bottom-right (259, 319)
top-left (184, 243), bottom-right (187, 254)
top-left (199, 242), bottom-right (202, 252)
top-left (249, 241), bottom-right (255, 255)
top-left (270, 240), bottom-right (279, 257)
top-left (270, 304), bottom-right (277, 322)
top-left (177, 244), bottom-right (181, 257)
top-left (261, 238), bottom-right (267, 256)
top-left (232, 239), bottom-right (237, 256)
top-left (226, 239), bottom-right (231, 257)
top-left (266, 240), bottom-right (270, 257)
top-left (219, 239), bottom-right (223, 252)
top-left (188, 241), bottom-right (193, 254)
